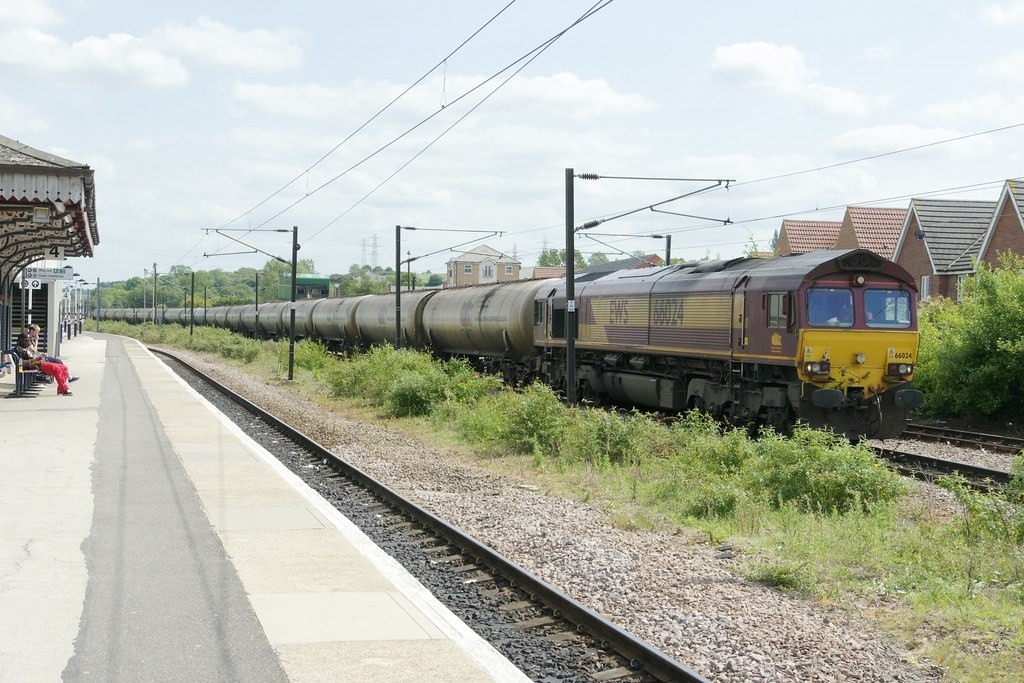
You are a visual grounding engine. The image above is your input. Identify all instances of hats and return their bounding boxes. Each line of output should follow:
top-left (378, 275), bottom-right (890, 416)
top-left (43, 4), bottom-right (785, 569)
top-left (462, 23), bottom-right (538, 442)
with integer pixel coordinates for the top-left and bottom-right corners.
top-left (18, 333), bottom-right (31, 341)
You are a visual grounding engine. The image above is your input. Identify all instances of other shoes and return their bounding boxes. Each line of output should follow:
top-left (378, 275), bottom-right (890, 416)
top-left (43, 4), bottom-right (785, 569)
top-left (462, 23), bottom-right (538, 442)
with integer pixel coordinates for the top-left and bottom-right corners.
top-left (68, 376), bottom-right (79, 383)
top-left (57, 390), bottom-right (73, 395)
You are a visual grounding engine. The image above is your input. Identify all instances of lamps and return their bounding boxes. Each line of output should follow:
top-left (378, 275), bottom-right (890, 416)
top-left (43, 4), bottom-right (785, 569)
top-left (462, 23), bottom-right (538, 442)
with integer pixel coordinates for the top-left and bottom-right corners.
top-left (913, 229), bottom-right (925, 241)
top-left (32, 206), bottom-right (50, 223)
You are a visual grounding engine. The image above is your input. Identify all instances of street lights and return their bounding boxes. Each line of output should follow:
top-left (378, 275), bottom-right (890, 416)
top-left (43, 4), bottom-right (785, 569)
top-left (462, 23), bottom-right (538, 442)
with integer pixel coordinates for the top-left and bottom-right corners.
top-left (64, 272), bottom-right (89, 340)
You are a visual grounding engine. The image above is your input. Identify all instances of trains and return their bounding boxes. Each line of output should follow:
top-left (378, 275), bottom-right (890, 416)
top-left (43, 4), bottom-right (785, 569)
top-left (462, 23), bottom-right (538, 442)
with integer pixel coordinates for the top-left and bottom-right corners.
top-left (92, 250), bottom-right (922, 444)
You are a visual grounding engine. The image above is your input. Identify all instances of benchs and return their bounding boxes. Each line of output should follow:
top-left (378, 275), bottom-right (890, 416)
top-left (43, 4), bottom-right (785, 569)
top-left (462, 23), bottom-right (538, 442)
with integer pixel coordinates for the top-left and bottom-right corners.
top-left (1, 349), bottom-right (46, 399)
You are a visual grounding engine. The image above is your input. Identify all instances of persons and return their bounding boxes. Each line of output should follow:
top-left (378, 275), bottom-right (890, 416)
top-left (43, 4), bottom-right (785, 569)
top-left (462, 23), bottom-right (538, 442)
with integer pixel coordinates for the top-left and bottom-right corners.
top-left (26, 323), bottom-right (80, 383)
top-left (14, 333), bottom-right (74, 396)
